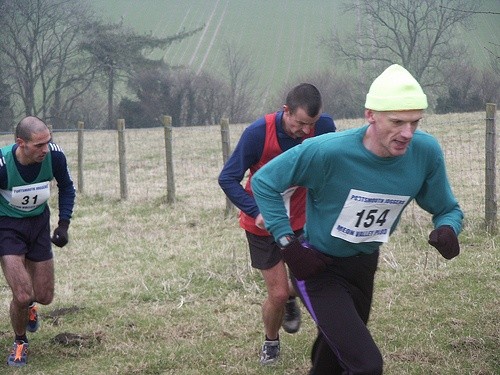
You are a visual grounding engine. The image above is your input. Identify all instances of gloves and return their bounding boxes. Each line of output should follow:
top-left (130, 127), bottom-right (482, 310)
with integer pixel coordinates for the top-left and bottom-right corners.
top-left (426, 226), bottom-right (459, 259)
top-left (281, 240), bottom-right (331, 280)
top-left (51, 220), bottom-right (70, 248)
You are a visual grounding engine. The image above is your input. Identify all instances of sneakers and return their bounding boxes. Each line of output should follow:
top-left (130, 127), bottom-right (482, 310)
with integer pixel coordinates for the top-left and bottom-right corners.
top-left (261, 334), bottom-right (280, 365)
top-left (26, 303), bottom-right (39, 333)
top-left (281, 296), bottom-right (302, 333)
top-left (8, 335), bottom-right (28, 366)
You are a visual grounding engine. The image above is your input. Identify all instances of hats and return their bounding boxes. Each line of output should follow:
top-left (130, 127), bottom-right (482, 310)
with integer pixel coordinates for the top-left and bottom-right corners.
top-left (366, 64), bottom-right (428, 113)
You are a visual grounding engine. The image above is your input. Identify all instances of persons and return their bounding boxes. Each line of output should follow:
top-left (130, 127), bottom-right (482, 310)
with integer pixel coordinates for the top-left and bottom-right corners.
top-left (249, 62), bottom-right (466, 375)
top-left (217, 82), bottom-right (338, 366)
top-left (1, 115), bottom-right (76, 370)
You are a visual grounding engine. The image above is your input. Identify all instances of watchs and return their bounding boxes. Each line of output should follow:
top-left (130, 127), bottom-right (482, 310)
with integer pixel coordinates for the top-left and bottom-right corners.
top-left (278, 235), bottom-right (297, 250)
